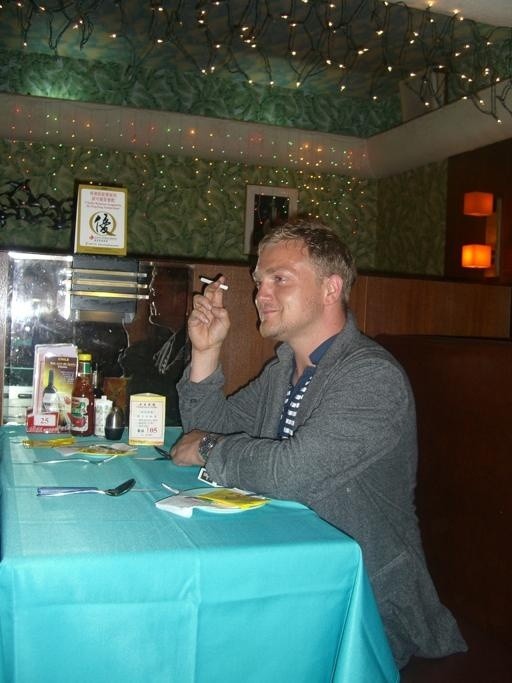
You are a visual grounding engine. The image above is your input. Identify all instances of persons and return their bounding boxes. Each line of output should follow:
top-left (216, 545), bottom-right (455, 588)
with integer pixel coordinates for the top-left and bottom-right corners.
top-left (171, 219), bottom-right (468, 671)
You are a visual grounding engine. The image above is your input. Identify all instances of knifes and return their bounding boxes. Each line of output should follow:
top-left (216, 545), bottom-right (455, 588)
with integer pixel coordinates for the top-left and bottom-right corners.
top-left (134, 456), bottom-right (171, 460)
top-left (153, 444), bottom-right (171, 456)
top-left (36, 487), bottom-right (160, 493)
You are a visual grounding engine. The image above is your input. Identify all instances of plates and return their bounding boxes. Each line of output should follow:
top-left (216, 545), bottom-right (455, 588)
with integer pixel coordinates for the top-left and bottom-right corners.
top-left (178, 488), bottom-right (269, 515)
top-left (78, 445), bottom-right (140, 458)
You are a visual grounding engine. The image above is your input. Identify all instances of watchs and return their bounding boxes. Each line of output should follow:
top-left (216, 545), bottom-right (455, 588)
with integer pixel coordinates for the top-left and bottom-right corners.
top-left (200, 434), bottom-right (223, 461)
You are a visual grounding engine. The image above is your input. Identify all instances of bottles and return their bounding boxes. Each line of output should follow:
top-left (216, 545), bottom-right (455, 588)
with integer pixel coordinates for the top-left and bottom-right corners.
top-left (105, 406), bottom-right (125, 440)
top-left (92, 362), bottom-right (104, 401)
top-left (94, 395), bottom-right (113, 437)
top-left (69, 355), bottom-right (96, 436)
top-left (43, 370), bottom-right (60, 415)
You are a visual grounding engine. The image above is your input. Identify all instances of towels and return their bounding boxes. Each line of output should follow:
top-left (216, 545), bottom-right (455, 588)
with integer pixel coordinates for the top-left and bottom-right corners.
top-left (57, 441), bottom-right (136, 457)
top-left (154, 489), bottom-right (272, 518)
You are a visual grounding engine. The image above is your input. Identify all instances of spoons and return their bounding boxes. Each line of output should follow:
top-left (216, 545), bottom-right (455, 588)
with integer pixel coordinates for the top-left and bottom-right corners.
top-left (36, 478), bottom-right (136, 496)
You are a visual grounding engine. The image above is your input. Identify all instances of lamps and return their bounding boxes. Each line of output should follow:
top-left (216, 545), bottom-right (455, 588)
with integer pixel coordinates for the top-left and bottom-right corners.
top-left (460, 192), bottom-right (501, 278)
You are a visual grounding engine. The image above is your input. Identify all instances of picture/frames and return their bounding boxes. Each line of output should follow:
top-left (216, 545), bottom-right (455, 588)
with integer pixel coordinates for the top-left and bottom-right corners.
top-left (246, 185), bottom-right (298, 254)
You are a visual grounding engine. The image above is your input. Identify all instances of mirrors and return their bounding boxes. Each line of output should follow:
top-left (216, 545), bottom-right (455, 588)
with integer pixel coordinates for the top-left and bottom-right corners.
top-left (2, 257), bottom-right (192, 425)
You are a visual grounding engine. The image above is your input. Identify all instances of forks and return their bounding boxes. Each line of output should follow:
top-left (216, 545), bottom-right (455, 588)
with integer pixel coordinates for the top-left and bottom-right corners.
top-left (33, 452), bottom-right (119, 465)
